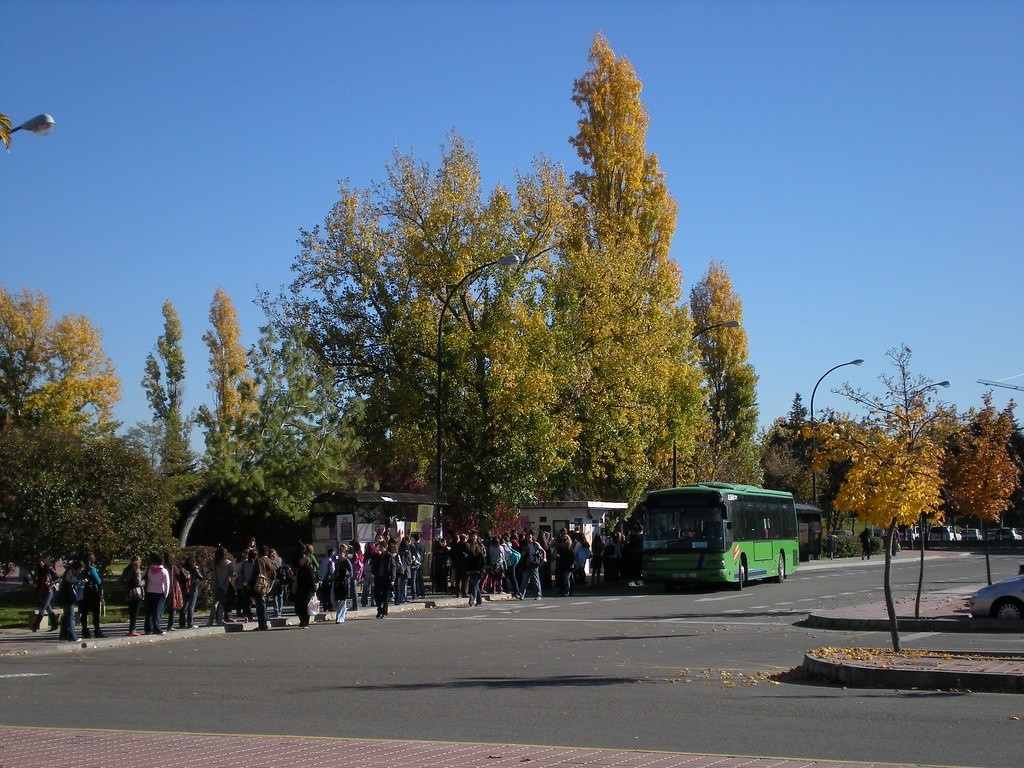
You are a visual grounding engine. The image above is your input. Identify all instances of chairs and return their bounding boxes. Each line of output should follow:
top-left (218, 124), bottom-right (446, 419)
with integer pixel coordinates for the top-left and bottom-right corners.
top-left (705, 521), bottom-right (722, 539)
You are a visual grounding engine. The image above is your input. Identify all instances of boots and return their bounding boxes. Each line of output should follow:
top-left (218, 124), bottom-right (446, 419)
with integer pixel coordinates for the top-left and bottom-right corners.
top-left (48, 612), bottom-right (58, 632)
top-left (29, 614), bottom-right (42, 632)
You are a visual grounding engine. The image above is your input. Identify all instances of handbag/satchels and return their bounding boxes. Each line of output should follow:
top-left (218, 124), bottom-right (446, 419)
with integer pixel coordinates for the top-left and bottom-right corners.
top-left (307, 595), bottom-right (320, 616)
top-left (255, 574), bottom-right (267, 595)
top-left (531, 551), bottom-right (546, 566)
top-left (85, 577), bottom-right (99, 592)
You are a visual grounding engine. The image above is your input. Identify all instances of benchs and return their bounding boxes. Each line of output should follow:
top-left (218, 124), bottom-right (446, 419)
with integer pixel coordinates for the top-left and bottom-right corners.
top-left (19, 607), bottom-right (79, 632)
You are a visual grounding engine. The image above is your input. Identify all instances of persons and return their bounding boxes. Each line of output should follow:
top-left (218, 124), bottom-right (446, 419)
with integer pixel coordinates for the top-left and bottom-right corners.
top-left (858, 528), bottom-right (871, 560)
top-left (892, 529), bottom-right (900, 556)
top-left (32, 530), bottom-right (643, 642)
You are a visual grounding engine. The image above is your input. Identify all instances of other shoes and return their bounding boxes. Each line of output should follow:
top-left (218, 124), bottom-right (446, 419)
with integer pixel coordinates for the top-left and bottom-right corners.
top-left (534, 596), bottom-right (541, 600)
top-left (474, 600), bottom-right (482, 606)
top-left (468, 600), bottom-right (473, 606)
top-left (516, 595), bottom-right (524, 600)
top-left (128, 632), bottom-right (141, 636)
top-left (498, 592), bottom-right (506, 594)
top-left (189, 625), bottom-right (199, 629)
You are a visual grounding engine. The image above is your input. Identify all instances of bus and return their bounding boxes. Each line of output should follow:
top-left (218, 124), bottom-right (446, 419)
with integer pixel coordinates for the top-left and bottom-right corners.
top-left (632, 482), bottom-right (800, 590)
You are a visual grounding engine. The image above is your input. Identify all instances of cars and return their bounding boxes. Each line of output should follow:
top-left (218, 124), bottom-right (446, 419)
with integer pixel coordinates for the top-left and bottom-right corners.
top-left (829, 525), bottom-right (1024, 542)
top-left (968, 573), bottom-right (1024, 618)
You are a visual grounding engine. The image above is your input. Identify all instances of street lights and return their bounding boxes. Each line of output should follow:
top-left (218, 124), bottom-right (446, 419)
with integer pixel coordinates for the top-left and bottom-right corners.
top-left (811, 359), bottom-right (864, 503)
top-left (671, 320), bottom-right (741, 486)
top-left (906, 380), bottom-right (951, 549)
top-left (432, 255), bottom-right (521, 595)
